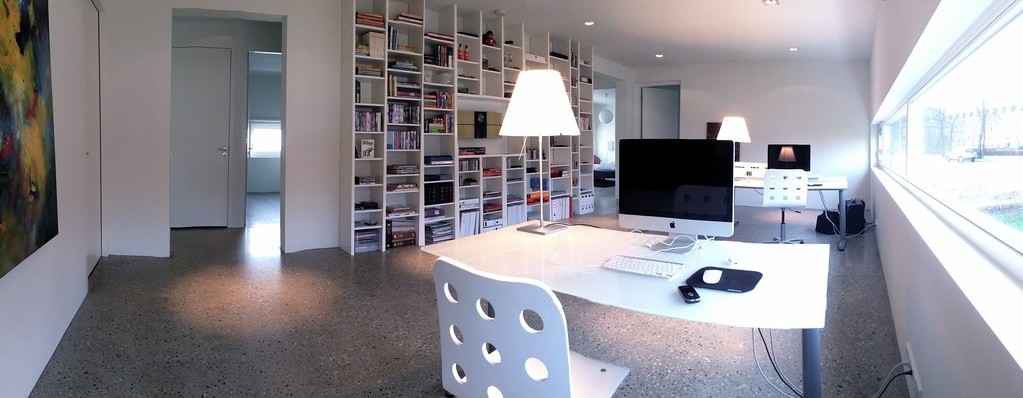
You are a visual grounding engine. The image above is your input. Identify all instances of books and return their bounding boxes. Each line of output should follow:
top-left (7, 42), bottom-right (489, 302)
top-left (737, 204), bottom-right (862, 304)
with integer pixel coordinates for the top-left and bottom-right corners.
top-left (353, 11), bottom-right (594, 255)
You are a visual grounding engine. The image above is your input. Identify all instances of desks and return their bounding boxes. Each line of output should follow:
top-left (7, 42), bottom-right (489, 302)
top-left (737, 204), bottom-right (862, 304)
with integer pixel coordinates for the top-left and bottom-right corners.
top-left (733, 176), bottom-right (848, 251)
top-left (418, 219), bottom-right (829, 398)
top-left (593, 170), bottom-right (616, 188)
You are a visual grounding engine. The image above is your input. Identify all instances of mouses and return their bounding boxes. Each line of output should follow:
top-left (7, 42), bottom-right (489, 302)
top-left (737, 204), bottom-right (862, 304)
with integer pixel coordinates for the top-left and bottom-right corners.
top-left (702, 270), bottom-right (722, 284)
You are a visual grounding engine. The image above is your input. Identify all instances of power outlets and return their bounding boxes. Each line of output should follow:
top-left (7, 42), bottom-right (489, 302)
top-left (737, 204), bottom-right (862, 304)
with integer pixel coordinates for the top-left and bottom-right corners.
top-left (903, 341), bottom-right (922, 395)
top-left (874, 218), bottom-right (880, 231)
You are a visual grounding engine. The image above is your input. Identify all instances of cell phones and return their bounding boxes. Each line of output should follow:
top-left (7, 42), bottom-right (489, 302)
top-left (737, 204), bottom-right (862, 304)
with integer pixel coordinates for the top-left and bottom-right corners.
top-left (677, 286), bottom-right (701, 304)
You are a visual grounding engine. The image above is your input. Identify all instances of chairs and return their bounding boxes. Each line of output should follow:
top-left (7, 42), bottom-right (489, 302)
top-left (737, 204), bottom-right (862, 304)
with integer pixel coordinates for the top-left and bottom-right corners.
top-left (431, 256), bottom-right (631, 398)
top-left (756, 169), bottom-right (809, 244)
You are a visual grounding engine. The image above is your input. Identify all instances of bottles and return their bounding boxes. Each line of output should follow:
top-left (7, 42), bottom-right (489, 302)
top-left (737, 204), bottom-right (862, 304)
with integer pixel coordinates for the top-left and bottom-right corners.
top-left (464, 45), bottom-right (470, 60)
top-left (458, 43), bottom-right (463, 59)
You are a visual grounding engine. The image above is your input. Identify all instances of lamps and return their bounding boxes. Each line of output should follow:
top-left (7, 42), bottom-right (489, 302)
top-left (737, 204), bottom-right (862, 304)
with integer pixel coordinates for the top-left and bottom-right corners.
top-left (498, 69), bottom-right (581, 236)
top-left (716, 116), bottom-right (751, 182)
top-left (598, 94), bottom-right (613, 124)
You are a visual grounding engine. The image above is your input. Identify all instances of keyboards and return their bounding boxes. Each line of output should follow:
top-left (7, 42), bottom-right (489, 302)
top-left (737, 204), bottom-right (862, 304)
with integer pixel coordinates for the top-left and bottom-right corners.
top-left (600, 254), bottom-right (685, 279)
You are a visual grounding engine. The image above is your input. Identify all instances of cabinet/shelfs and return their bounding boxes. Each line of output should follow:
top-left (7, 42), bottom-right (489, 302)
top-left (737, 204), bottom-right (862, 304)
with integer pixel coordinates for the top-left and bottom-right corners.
top-left (338, 0), bottom-right (594, 256)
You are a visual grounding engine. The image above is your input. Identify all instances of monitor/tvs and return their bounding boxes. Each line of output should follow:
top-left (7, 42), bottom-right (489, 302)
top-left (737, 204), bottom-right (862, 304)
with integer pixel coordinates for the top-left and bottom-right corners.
top-left (619, 138), bottom-right (735, 254)
top-left (767, 144), bottom-right (812, 172)
